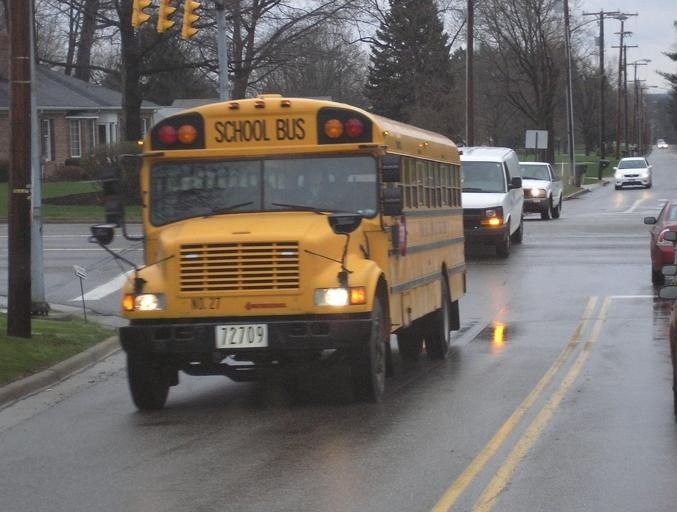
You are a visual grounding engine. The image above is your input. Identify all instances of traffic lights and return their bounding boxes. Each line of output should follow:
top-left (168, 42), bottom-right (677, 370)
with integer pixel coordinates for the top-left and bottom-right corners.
top-left (131, 0), bottom-right (201, 41)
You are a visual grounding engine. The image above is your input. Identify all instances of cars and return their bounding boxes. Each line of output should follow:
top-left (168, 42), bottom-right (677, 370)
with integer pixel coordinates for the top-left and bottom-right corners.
top-left (612, 156), bottom-right (653, 190)
top-left (644, 199), bottom-right (677, 287)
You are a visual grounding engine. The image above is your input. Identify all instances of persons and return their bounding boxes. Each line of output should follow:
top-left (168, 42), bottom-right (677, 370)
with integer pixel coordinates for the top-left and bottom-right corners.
top-left (290, 169), bottom-right (335, 210)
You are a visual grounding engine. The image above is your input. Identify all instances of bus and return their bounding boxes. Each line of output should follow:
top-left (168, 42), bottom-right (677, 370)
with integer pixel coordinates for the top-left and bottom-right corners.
top-left (88, 94), bottom-right (467, 411)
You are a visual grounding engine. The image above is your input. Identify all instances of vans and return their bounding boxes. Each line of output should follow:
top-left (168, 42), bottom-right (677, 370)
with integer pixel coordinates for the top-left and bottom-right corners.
top-left (457, 146), bottom-right (524, 258)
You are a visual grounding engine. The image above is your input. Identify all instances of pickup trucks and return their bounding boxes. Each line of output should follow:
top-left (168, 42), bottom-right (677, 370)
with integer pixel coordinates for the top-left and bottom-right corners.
top-left (518, 161), bottom-right (565, 219)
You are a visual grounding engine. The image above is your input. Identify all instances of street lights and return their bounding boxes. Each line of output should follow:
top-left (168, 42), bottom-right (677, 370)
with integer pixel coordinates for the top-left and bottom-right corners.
top-left (564, 7), bottom-right (665, 184)
